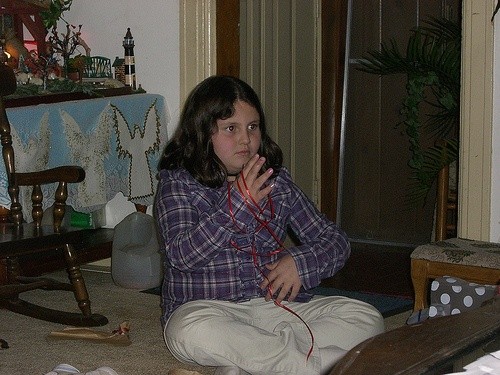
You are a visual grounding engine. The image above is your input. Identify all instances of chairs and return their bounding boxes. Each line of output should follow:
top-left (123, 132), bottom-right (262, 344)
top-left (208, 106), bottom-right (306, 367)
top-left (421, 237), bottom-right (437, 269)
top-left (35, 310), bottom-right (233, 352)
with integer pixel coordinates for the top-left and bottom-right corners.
top-left (0, 63), bottom-right (114, 350)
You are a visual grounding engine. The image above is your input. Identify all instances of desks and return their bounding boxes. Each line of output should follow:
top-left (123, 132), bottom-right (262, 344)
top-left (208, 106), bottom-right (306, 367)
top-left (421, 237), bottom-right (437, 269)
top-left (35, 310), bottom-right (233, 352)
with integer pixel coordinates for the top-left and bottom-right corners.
top-left (329, 300), bottom-right (500, 375)
top-left (0, 94), bottom-right (171, 214)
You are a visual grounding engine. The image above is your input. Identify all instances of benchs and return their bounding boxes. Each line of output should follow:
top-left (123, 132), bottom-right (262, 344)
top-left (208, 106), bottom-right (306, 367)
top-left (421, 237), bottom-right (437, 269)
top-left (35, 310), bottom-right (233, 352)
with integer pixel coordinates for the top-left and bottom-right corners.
top-left (82, 57), bottom-right (111, 83)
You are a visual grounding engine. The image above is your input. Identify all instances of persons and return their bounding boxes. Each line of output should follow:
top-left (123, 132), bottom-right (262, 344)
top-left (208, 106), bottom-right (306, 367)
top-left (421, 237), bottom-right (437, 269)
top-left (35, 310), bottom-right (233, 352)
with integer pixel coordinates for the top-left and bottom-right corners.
top-left (152, 74), bottom-right (385, 375)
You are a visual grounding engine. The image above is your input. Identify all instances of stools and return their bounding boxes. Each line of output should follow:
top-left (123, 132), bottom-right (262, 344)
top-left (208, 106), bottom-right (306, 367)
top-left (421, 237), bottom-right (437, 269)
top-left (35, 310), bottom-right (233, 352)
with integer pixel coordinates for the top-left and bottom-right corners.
top-left (411, 237), bottom-right (500, 311)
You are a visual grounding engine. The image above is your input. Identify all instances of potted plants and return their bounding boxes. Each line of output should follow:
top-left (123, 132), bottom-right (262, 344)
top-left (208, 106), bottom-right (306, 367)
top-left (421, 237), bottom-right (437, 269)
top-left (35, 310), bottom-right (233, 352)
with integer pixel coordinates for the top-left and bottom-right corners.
top-left (67, 54), bottom-right (85, 80)
